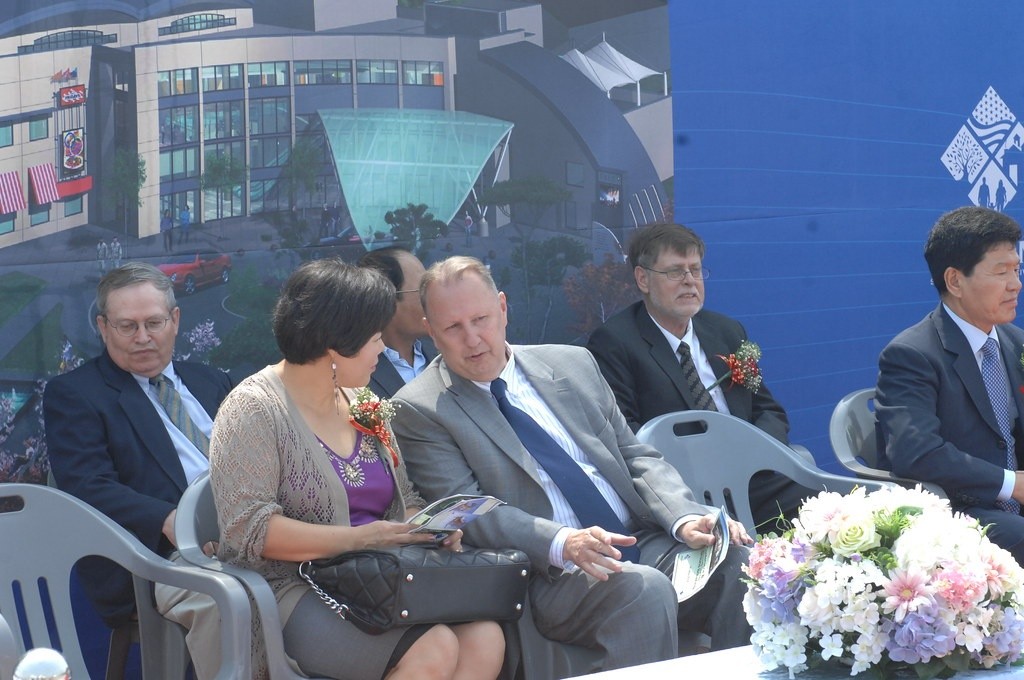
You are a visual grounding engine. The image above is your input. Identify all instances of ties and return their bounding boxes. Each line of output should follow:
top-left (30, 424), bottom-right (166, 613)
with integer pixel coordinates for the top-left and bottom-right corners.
top-left (491, 378), bottom-right (641, 564)
top-left (149, 374), bottom-right (209, 458)
top-left (678, 341), bottom-right (718, 412)
top-left (981, 338), bottom-right (1020, 515)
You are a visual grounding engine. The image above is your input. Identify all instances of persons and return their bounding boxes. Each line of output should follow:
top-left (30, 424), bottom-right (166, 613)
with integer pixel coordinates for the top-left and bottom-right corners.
top-left (363, 246), bottom-right (439, 403)
top-left (210, 259), bottom-right (505, 680)
top-left (43, 261), bottom-right (238, 680)
top-left (875, 207), bottom-right (1024, 572)
top-left (587, 220), bottom-right (821, 541)
top-left (385, 256), bottom-right (755, 673)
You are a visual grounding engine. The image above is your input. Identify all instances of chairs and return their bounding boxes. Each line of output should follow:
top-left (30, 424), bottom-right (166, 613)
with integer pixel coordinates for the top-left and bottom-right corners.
top-left (829, 387), bottom-right (949, 505)
top-left (0, 481), bottom-right (254, 680)
top-left (633, 410), bottom-right (889, 547)
top-left (176, 469), bottom-right (317, 680)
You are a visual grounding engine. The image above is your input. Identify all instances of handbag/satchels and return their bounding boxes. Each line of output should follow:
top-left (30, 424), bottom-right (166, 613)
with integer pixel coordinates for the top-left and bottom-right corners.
top-left (296, 542), bottom-right (531, 635)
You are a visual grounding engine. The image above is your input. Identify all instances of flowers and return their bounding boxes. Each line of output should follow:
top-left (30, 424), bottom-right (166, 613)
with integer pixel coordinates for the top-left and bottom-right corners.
top-left (698, 342), bottom-right (761, 397)
top-left (735, 484), bottom-right (1023, 680)
top-left (350, 387), bottom-right (403, 433)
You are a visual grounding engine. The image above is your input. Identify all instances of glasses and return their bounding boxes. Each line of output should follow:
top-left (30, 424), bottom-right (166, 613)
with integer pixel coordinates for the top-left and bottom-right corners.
top-left (105, 315), bottom-right (170, 337)
top-left (641, 265), bottom-right (710, 281)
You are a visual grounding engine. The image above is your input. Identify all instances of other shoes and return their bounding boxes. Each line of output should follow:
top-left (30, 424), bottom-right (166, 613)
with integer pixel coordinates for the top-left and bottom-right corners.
top-left (178, 240), bottom-right (181, 244)
top-left (185, 239), bottom-right (188, 243)
top-left (165, 248), bottom-right (173, 252)
